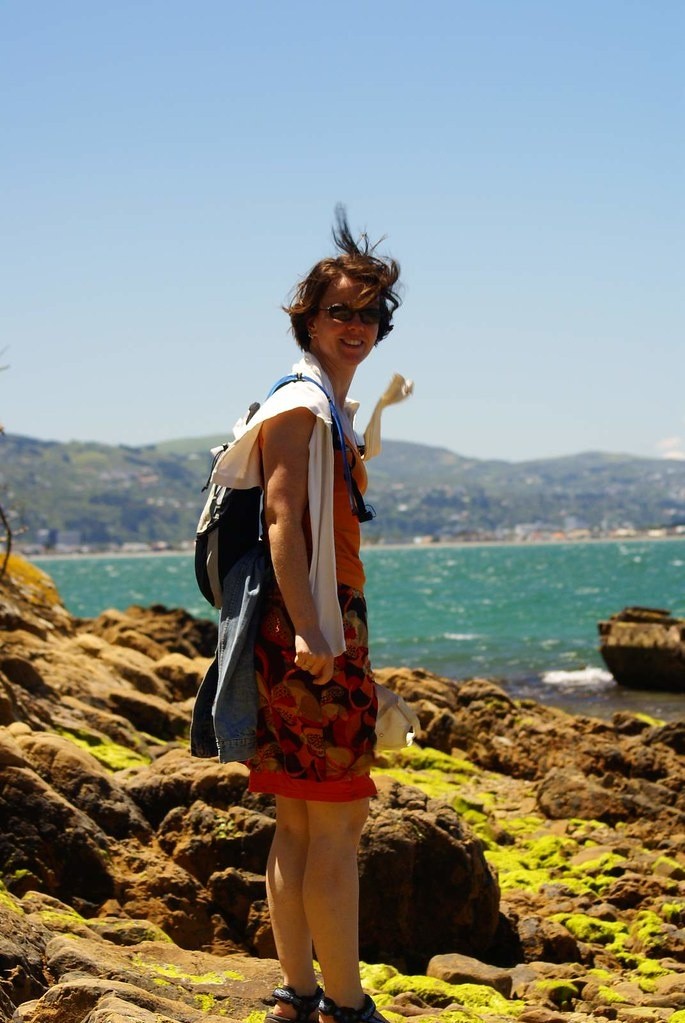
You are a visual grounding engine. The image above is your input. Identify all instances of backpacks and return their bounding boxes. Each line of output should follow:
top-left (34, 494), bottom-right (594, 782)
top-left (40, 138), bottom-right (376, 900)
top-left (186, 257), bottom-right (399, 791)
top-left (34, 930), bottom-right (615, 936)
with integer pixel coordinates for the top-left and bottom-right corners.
top-left (194, 373), bottom-right (355, 609)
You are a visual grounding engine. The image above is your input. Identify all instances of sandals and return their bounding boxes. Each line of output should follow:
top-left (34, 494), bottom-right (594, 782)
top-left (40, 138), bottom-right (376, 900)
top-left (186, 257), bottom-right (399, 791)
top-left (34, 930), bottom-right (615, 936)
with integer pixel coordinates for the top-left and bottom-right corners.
top-left (318, 994), bottom-right (391, 1023)
top-left (264, 985), bottom-right (324, 1023)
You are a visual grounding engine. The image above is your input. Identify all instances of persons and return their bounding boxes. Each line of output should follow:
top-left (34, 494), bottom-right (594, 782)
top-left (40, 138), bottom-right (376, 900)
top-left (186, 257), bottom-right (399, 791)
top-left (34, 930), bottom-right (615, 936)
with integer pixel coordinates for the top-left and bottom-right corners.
top-left (188, 203), bottom-right (413, 1023)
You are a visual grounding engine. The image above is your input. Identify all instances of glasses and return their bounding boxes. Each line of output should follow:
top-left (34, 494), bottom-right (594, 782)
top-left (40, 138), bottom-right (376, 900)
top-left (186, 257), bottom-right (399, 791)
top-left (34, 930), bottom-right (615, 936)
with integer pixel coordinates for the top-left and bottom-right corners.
top-left (318, 302), bottom-right (381, 325)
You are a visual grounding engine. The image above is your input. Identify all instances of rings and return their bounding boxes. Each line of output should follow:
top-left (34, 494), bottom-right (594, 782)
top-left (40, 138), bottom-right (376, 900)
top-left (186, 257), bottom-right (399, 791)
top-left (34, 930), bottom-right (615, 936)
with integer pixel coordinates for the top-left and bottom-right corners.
top-left (304, 663), bottom-right (311, 668)
